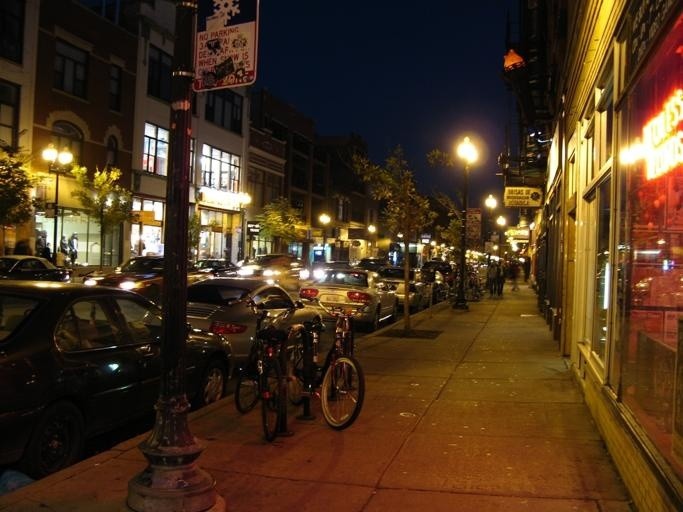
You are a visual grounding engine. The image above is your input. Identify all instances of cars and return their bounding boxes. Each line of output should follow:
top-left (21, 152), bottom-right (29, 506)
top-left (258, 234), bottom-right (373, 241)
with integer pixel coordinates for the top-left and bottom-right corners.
top-left (154, 269), bottom-right (328, 410)
top-left (0, 279), bottom-right (238, 479)
top-left (295, 267), bottom-right (407, 335)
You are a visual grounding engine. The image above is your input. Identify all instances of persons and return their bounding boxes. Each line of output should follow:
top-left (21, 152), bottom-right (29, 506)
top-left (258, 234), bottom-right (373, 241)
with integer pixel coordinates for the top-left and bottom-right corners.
top-left (487, 257), bottom-right (531, 298)
top-left (59, 232), bottom-right (78, 265)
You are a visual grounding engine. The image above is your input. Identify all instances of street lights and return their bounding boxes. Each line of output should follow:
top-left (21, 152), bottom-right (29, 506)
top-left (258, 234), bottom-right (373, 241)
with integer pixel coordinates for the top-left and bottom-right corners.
top-left (318, 212), bottom-right (333, 263)
top-left (366, 223), bottom-right (377, 259)
top-left (452, 135), bottom-right (484, 314)
top-left (494, 215), bottom-right (507, 270)
top-left (235, 192), bottom-right (253, 263)
top-left (40, 141), bottom-right (78, 266)
top-left (483, 194), bottom-right (498, 292)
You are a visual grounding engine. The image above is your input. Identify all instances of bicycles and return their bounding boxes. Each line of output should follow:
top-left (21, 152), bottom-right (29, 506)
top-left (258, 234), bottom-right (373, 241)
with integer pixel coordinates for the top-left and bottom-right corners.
top-left (222, 289), bottom-right (367, 442)
